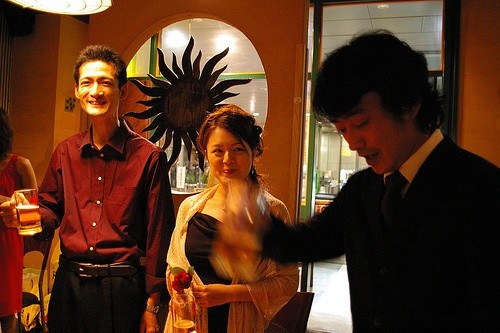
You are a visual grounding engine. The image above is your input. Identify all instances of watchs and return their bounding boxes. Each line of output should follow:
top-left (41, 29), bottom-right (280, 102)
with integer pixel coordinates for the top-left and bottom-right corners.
top-left (145, 302), bottom-right (160, 314)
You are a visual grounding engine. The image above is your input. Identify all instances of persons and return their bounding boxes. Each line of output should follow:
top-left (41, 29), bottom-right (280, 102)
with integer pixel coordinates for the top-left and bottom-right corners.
top-left (163, 104), bottom-right (300, 333)
top-left (214, 28), bottom-right (500, 333)
top-left (0, 45), bottom-right (176, 333)
top-left (0, 106), bottom-right (40, 333)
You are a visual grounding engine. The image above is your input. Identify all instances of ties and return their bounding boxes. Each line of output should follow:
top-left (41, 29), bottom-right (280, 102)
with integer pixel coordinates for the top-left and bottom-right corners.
top-left (381, 172), bottom-right (408, 227)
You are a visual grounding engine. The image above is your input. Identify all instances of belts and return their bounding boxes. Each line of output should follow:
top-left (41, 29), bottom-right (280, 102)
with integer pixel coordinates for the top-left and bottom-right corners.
top-left (59, 255), bottom-right (137, 277)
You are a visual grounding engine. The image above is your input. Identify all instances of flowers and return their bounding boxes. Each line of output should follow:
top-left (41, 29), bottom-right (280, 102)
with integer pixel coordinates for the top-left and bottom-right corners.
top-left (166, 264), bottom-right (197, 296)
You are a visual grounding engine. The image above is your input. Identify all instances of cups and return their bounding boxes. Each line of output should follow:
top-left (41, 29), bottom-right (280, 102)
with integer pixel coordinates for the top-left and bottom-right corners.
top-left (170, 294), bottom-right (197, 333)
top-left (14, 188), bottom-right (42, 236)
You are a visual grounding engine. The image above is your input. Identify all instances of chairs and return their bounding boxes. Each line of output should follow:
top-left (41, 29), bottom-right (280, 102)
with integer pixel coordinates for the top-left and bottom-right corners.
top-left (263, 291), bottom-right (315, 333)
